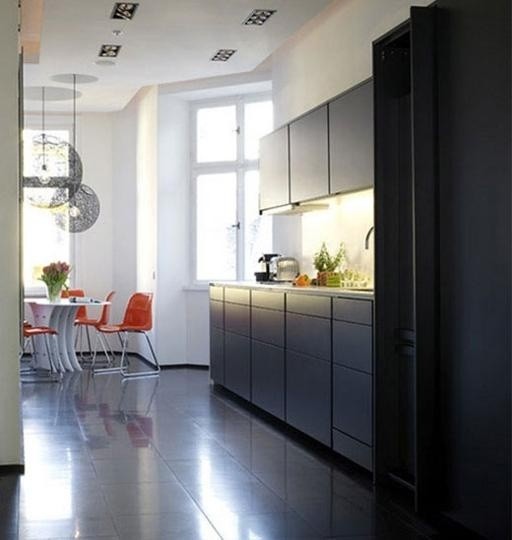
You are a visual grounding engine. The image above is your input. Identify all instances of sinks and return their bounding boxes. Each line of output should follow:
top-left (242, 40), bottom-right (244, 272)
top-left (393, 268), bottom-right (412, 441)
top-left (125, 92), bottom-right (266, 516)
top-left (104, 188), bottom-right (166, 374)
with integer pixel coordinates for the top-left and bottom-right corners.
top-left (336, 288), bottom-right (373, 292)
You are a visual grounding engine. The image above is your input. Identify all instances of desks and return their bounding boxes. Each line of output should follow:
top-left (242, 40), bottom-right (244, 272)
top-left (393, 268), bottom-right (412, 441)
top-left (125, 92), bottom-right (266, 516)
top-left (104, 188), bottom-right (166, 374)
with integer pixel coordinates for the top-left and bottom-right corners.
top-left (24, 297), bottom-right (111, 373)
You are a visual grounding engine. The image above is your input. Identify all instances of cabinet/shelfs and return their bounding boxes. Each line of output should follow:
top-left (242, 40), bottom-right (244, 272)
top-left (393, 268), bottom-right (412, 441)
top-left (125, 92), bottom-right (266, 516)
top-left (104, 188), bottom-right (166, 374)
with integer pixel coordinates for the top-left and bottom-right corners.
top-left (258, 76), bottom-right (374, 215)
top-left (209, 286), bottom-right (374, 473)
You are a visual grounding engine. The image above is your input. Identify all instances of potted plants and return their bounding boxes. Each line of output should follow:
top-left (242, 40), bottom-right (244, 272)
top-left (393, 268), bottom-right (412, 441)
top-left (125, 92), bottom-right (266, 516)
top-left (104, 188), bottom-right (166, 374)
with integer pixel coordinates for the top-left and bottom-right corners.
top-left (312, 241), bottom-right (346, 287)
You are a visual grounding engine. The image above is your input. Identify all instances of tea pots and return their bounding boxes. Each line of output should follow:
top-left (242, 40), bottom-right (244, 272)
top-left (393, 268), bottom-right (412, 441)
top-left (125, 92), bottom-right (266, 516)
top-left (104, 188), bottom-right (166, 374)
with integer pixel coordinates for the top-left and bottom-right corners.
top-left (254, 254), bottom-right (282, 282)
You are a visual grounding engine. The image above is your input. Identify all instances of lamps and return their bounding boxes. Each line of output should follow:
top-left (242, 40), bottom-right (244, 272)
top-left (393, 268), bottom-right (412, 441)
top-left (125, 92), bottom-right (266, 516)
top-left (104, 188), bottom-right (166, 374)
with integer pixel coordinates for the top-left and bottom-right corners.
top-left (23, 86), bottom-right (83, 208)
top-left (49, 74), bottom-right (100, 233)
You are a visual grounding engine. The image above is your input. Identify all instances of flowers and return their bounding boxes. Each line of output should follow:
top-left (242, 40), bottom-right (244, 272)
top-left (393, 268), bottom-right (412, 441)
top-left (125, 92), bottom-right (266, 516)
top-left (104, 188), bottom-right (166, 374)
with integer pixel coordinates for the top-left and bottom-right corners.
top-left (37, 262), bottom-right (73, 300)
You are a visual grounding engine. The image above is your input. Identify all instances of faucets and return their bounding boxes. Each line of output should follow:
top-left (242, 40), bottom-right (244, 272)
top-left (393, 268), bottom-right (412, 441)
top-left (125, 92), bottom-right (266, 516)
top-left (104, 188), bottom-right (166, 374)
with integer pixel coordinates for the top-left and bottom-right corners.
top-left (364, 225), bottom-right (374, 249)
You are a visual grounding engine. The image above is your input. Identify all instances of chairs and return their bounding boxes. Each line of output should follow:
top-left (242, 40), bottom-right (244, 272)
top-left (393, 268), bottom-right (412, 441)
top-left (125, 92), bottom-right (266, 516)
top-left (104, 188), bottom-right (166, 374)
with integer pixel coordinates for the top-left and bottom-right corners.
top-left (75, 366), bottom-right (114, 436)
top-left (20, 326), bottom-right (63, 383)
top-left (73, 291), bottom-right (116, 368)
top-left (91, 292), bottom-right (161, 377)
top-left (61, 290), bottom-right (93, 361)
top-left (20, 320), bottom-right (37, 376)
top-left (98, 370), bottom-right (160, 448)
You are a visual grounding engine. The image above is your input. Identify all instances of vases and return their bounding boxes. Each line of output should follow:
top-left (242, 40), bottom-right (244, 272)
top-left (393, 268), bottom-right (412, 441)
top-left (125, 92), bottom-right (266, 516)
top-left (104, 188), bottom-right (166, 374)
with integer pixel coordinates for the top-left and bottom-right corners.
top-left (47, 285), bottom-right (62, 302)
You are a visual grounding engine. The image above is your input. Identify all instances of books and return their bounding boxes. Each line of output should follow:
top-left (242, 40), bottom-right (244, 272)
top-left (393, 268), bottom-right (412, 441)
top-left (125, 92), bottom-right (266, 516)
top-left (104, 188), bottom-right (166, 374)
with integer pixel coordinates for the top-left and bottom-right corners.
top-left (69, 295), bottom-right (102, 303)
